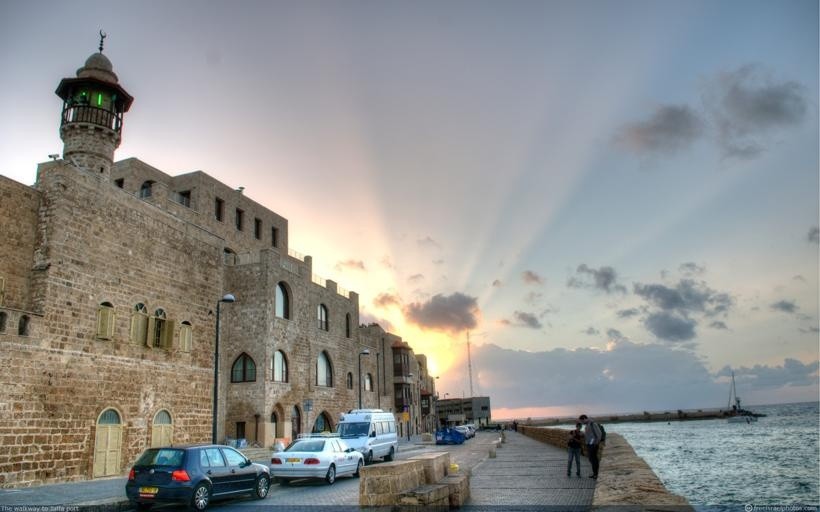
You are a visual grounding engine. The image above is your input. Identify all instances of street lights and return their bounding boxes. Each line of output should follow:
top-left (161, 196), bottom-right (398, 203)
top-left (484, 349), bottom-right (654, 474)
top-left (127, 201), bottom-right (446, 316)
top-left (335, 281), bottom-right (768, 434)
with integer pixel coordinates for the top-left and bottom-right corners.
top-left (443, 392), bottom-right (450, 424)
top-left (358, 349), bottom-right (370, 409)
top-left (212, 293), bottom-right (235, 445)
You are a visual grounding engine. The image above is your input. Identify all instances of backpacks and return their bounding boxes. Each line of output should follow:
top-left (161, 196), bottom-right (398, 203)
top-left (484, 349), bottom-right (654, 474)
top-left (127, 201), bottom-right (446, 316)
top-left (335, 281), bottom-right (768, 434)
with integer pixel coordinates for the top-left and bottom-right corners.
top-left (590, 421), bottom-right (606, 443)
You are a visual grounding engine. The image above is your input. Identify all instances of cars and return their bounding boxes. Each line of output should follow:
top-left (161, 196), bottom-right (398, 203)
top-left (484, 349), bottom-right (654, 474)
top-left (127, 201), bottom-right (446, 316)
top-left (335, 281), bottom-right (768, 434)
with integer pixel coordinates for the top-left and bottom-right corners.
top-left (126, 437), bottom-right (365, 510)
top-left (454, 425), bottom-right (476, 440)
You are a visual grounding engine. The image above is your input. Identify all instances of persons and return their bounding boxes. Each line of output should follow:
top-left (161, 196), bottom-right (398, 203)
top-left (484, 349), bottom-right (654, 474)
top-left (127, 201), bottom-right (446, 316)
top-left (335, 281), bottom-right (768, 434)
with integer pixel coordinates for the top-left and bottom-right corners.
top-left (579, 416), bottom-right (602, 479)
top-left (567, 423), bottom-right (583, 476)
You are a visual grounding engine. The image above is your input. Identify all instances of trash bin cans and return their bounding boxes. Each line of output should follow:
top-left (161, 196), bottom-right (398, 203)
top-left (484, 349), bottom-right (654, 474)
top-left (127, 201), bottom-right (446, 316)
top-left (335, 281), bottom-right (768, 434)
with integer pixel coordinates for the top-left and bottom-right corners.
top-left (227, 439), bottom-right (248, 449)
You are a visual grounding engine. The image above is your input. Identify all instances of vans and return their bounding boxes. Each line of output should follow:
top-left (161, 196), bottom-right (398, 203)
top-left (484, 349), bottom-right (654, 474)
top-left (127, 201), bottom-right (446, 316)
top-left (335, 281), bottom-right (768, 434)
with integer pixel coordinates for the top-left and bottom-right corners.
top-left (336, 409), bottom-right (398, 466)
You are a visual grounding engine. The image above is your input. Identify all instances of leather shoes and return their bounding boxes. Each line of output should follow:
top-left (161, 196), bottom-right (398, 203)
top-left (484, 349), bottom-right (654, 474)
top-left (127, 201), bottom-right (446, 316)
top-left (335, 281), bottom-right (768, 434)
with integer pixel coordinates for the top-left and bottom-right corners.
top-left (588, 474), bottom-right (597, 480)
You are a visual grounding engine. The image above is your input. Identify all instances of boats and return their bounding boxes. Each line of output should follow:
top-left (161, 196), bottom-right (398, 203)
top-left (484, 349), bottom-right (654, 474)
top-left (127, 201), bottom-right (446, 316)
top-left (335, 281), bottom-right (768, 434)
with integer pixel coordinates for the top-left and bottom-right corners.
top-left (728, 370), bottom-right (757, 422)
